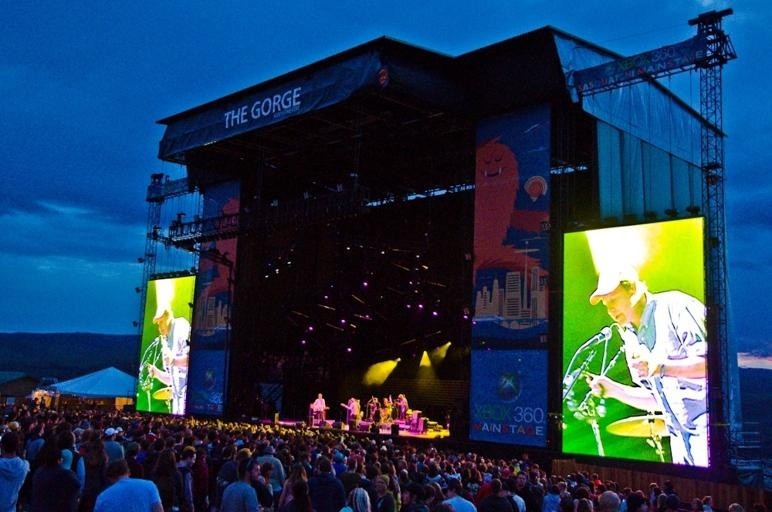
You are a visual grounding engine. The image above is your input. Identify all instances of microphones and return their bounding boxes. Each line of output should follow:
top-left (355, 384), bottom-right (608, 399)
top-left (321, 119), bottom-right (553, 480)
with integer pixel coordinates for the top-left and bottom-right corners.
top-left (576, 327), bottom-right (612, 354)
top-left (154, 335), bottom-right (162, 342)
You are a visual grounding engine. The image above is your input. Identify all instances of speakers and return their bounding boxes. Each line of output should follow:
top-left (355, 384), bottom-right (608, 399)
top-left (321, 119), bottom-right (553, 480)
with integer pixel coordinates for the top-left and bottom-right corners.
top-left (306, 416), bottom-right (314, 426)
top-left (359, 424), bottom-right (371, 431)
top-left (370, 428), bottom-right (379, 433)
top-left (333, 422), bottom-right (345, 429)
top-left (391, 424), bottom-right (399, 435)
top-left (319, 421), bottom-right (331, 429)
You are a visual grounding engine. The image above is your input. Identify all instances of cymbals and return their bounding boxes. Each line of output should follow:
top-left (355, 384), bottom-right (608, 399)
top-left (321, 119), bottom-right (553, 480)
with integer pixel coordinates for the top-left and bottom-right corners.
top-left (153, 387), bottom-right (172, 400)
top-left (608, 415), bottom-right (668, 438)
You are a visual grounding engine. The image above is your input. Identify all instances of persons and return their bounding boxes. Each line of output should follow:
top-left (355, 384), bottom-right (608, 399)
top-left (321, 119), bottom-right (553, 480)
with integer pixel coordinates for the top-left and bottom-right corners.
top-left (146, 305), bottom-right (191, 416)
top-left (584, 263), bottom-right (708, 468)
top-left (1, 393), bottom-right (772, 512)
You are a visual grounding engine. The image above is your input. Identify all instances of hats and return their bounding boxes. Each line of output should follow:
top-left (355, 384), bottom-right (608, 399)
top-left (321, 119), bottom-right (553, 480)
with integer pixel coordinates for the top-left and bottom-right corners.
top-left (589, 265), bottom-right (639, 306)
top-left (153, 303), bottom-right (171, 323)
top-left (105, 427), bottom-right (118, 436)
